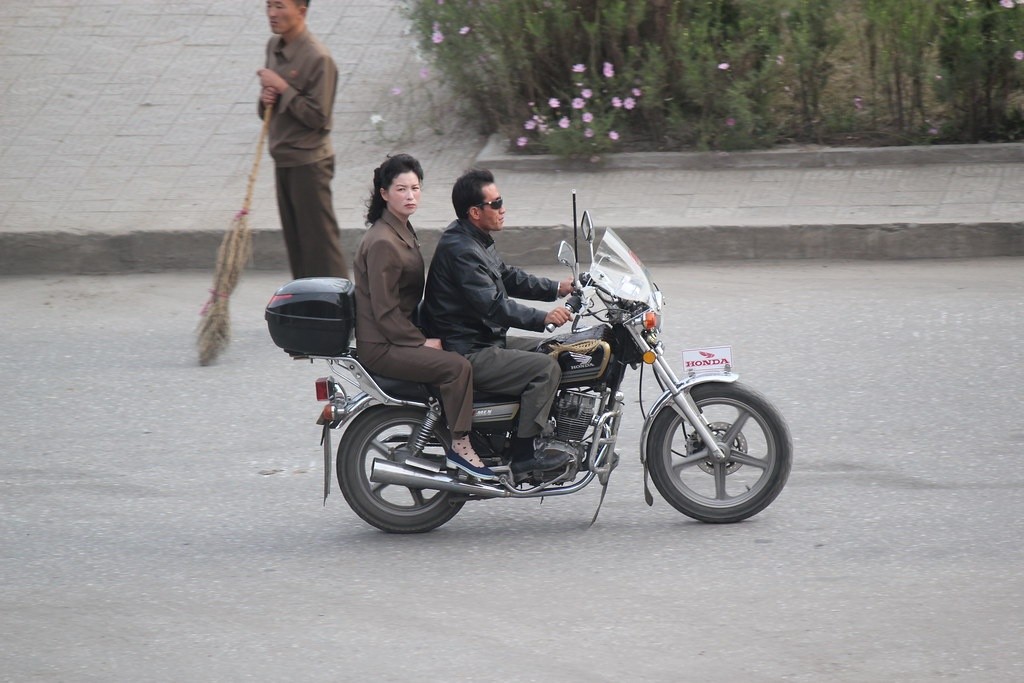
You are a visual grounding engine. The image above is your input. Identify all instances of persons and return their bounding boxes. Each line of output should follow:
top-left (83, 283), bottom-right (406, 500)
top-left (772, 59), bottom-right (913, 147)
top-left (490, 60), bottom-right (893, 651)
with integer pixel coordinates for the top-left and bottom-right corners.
top-left (420, 169), bottom-right (574, 483)
top-left (352, 153), bottom-right (494, 481)
top-left (257, 0), bottom-right (349, 280)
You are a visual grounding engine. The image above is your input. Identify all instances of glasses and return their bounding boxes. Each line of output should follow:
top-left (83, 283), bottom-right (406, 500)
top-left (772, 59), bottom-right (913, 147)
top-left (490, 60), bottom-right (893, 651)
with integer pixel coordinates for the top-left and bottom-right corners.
top-left (466, 198), bottom-right (502, 214)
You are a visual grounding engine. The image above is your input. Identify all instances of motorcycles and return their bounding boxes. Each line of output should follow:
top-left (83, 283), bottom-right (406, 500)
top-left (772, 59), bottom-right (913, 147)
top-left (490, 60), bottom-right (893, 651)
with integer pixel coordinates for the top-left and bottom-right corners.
top-left (265, 190), bottom-right (795, 533)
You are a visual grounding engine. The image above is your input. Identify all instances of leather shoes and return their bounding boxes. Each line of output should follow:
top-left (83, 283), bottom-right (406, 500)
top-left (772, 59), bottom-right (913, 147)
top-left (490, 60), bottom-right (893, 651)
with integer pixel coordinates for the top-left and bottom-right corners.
top-left (511, 448), bottom-right (571, 482)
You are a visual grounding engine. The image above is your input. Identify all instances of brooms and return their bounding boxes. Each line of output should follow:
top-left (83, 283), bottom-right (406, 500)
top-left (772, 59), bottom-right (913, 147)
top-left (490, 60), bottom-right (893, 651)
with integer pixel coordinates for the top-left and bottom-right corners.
top-left (194, 102), bottom-right (280, 370)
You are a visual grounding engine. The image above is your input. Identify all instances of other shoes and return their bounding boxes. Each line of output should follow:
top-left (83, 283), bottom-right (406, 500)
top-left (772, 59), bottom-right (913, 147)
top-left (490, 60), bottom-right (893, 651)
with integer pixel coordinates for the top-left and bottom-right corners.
top-left (445, 448), bottom-right (495, 481)
